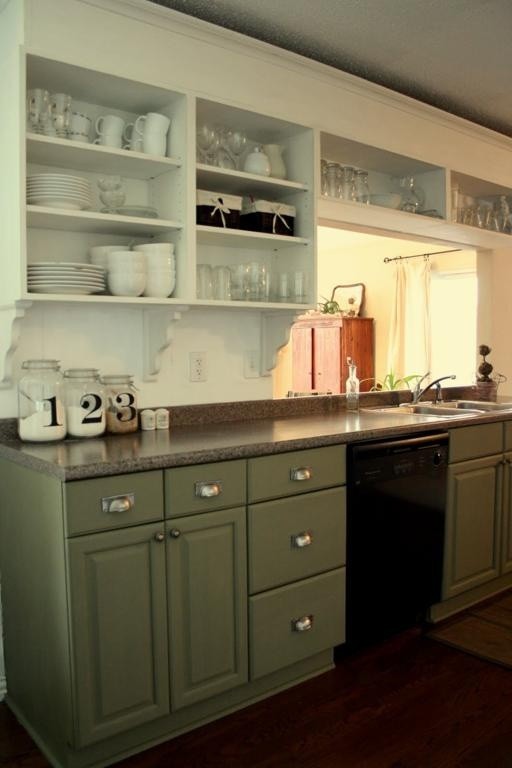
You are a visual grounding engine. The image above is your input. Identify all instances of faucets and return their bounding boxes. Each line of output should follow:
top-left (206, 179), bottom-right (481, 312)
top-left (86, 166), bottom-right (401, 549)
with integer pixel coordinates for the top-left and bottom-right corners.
top-left (412, 373), bottom-right (456, 404)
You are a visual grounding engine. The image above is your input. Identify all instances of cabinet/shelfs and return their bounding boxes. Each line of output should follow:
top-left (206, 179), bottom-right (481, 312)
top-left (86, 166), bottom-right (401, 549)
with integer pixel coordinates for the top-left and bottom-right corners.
top-left (347, 420), bottom-right (512, 628)
top-left (291, 313), bottom-right (375, 395)
top-left (318, 57), bottom-right (512, 253)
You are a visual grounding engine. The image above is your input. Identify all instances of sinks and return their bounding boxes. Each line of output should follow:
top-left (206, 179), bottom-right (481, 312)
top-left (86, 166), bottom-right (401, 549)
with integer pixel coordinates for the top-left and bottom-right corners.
top-left (359, 404), bottom-right (491, 418)
top-left (421, 399), bottom-right (512, 412)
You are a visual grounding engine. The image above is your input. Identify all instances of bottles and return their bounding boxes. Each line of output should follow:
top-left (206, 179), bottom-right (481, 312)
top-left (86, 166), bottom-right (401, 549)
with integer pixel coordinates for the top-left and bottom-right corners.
top-left (244, 142), bottom-right (288, 179)
top-left (345, 364), bottom-right (361, 413)
top-left (17, 359), bottom-right (171, 443)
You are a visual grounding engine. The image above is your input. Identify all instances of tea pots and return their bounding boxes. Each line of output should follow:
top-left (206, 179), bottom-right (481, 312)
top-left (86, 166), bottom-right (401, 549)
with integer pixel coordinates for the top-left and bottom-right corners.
top-left (390, 173), bottom-right (425, 213)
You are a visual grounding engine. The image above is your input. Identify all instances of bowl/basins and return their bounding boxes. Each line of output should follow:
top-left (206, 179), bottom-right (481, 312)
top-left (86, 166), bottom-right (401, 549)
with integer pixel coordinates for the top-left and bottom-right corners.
top-left (89, 242), bottom-right (176, 297)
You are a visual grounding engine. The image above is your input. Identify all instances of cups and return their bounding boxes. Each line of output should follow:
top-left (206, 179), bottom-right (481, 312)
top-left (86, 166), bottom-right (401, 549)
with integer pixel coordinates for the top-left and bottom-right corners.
top-left (195, 260), bottom-right (308, 305)
top-left (321, 154), bottom-right (370, 207)
top-left (99, 174), bottom-right (127, 213)
top-left (28, 86), bottom-right (171, 157)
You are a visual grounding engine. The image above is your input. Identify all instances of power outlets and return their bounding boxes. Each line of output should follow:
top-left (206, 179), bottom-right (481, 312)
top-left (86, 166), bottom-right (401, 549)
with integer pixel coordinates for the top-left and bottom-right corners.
top-left (189, 352), bottom-right (208, 383)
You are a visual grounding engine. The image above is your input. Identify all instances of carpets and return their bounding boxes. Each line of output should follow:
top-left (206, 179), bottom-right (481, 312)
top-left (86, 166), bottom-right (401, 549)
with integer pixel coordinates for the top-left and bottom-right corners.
top-left (423, 590), bottom-right (512, 668)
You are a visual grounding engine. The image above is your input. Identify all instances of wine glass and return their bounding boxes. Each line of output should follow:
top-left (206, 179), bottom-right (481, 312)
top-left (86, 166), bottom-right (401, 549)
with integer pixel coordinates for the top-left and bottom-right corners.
top-left (195, 125), bottom-right (247, 171)
top-left (456, 183), bottom-right (512, 233)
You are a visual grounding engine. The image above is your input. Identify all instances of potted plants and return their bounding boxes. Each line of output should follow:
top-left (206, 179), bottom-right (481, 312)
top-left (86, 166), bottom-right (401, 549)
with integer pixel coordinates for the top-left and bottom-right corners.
top-left (475, 345), bottom-right (498, 402)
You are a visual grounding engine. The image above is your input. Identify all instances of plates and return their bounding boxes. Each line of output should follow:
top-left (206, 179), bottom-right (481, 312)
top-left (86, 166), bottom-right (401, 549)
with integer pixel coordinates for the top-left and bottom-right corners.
top-left (356, 193), bottom-right (403, 210)
top-left (27, 262), bottom-right (106, 295)
top-left (26, 173), bottom-right (94, 211)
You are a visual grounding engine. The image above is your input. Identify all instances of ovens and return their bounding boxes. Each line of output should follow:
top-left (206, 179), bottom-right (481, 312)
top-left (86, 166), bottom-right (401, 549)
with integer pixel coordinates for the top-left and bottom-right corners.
top-left (348, 431), bottom-right (449, 656)
